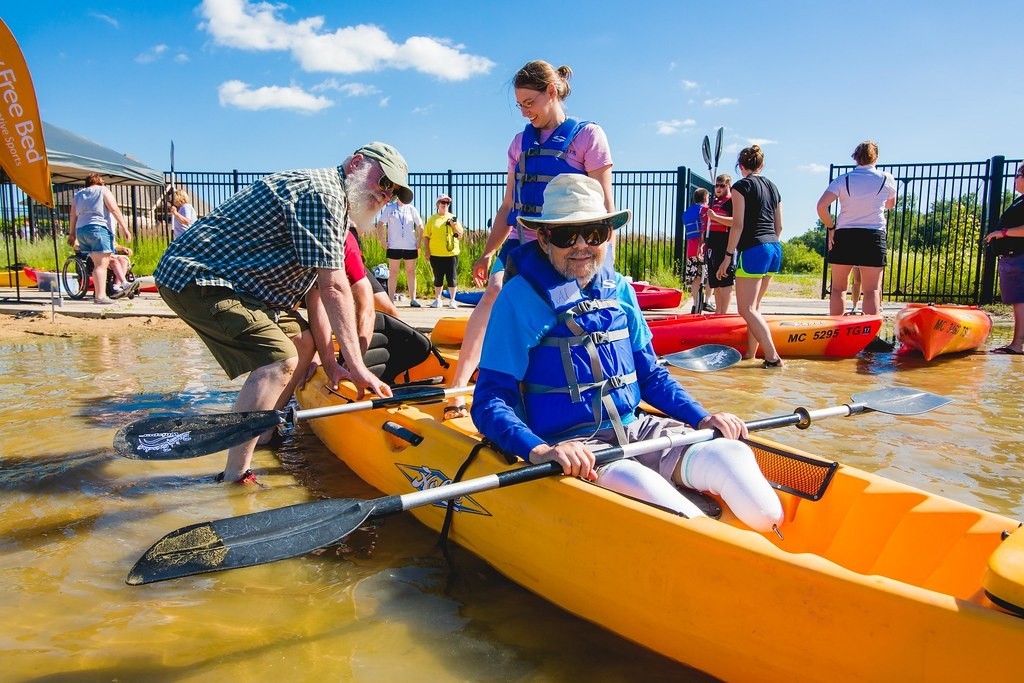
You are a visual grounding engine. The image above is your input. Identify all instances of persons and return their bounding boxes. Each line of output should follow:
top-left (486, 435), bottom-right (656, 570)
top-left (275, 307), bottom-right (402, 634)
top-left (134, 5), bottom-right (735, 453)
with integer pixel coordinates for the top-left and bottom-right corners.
top-left (169, 191), bottom-right (198, 240)
top-left (470, 174), bottom-right (784, 535)
top-left (377, 198), bottom-right (423, 308)
top-left (440, 59), bottom-right (615, 422)
top-left (67, 174), bottom-right (131, 304)
top-left (296, 230), bottom-right (399, 391)
top-left (985, 164), bottom-right (1024, 355)
top-left (152, 139), bottom-right (414, 489)
top-left (852, 267), bottom-right (883, 314)
top-left (707, 174), bottom-right (741, 315)
top-left (683, 188), bottom-right (716, 314)
top-left (15, 219), bottom-right (60, 244)
top-left (817, 140), bottom-right (897, 352)
top-left (423, 194), bottom-right (464, 309)
top-left (75, 240), bottom-right (140, 300)
top-left (716, 145), bottom-right (784, 369)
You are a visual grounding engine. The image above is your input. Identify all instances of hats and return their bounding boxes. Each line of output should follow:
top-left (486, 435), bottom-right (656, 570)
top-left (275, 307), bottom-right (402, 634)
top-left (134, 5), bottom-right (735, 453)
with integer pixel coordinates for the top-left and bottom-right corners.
top-left (435, 194), bottom-right (452, 207)
top-left (515, 173), bottom-right (632, 231)
top-left (353, 142), bottom-right (414, 205)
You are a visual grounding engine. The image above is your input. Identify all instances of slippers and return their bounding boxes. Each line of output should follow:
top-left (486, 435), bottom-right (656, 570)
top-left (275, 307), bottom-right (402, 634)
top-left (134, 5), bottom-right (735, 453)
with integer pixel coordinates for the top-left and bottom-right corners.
top-left (990, 344), bottom-right (1024, 355)
top-left (442, 404), bottom-right (470, 422)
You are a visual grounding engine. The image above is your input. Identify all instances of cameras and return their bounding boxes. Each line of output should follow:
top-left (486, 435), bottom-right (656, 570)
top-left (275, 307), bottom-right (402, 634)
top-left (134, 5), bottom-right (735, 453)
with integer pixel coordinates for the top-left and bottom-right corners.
top-left (446, 217), bottom-right (457, 225)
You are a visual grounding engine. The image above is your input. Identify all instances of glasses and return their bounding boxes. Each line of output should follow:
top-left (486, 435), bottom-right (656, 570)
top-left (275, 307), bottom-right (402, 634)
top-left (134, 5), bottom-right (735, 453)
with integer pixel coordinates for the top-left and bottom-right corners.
top-left (1015, 174), bottom-right (1023, 178)
top-left (367, 159), bottom-right (401, 204)
top-left (541, 220), bottom-right (614, 249)
top-left (515, 91), bottom-right (544, 109)
top-left (715, 183), bottom-right (728, 188)
top-left (439, 200), bottom-right (451, 206)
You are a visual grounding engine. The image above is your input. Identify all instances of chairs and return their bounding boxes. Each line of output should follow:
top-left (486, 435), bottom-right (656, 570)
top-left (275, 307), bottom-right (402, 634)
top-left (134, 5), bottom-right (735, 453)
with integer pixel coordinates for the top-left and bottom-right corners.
top-left (339, 310), bottom-right (444, 389)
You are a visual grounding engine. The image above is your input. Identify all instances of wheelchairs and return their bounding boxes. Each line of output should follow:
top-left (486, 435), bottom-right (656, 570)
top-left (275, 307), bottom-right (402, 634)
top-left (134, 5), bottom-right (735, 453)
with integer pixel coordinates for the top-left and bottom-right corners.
top-left (62, 246), bottom-right (143, 301)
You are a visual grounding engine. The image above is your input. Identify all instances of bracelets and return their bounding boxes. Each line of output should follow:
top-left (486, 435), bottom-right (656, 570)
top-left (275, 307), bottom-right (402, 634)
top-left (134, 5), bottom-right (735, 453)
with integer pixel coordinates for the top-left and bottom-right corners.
top-left (725, 251), bottom-right (734, 258)
top-left (827, 226), bottom-right (835, 231)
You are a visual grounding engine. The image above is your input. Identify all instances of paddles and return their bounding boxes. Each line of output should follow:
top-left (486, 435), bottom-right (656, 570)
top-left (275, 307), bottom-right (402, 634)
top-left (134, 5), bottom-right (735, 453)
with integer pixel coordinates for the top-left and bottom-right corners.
top-left (695, 126), bottom-right (724, 316)
top-left (112, 343), bottom-right (743, 462)
top-left (125, 385), bottom-right (954, 586)
top-left (170, 139), bottom-right (175, 244)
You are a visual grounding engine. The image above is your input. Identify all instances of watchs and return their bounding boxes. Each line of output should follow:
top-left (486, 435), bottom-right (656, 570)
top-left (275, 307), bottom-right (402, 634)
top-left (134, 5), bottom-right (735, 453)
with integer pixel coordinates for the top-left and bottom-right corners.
top-left (1001, 228), bottom-right (1007, 238)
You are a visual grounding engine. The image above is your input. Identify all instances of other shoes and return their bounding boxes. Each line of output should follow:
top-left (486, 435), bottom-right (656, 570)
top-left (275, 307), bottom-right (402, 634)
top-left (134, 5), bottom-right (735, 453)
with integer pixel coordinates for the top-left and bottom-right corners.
top-left (430, 298), bottom-right (443, 308)
top-left (702, 302), bottom-right (716, 312)
top-left (256, 426), bottom-right (303, 449)
top-left (864, 334), bottom-right (894, 352)
top-left (214, 469), bottom-right (272, 494)
top-left (123, 279), bottom-right (140, 297)
top-left (691, 305), bottom-right (699, 314)
top-left (763, 358), bottom-right (784, 368)
top-left (394, 293), bottom-right (399, 301)
top-left (94, 296), bottom-right (118, 305)
top-left (109, 288), bottom-right (126, 299)
top-left (399, 294), bottom-right (408, 301)
top-left (448, 299), bottom-right (458, 309)
top-left (410, 300), bottom-right (421, 307)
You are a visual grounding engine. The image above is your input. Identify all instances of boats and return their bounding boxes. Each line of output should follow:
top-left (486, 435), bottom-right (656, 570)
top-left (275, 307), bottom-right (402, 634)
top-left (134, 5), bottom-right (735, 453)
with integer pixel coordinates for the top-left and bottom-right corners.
top-left (655, 315), bottom-right (884, 360)
top-left (295, 311), bottom-right (1024, 682)
top-left (893, 301), bottom-right (993, 362)
top-left (24, 266), bottom-right (158, 293)
top-left (441, 282), bottom-right (683, 312)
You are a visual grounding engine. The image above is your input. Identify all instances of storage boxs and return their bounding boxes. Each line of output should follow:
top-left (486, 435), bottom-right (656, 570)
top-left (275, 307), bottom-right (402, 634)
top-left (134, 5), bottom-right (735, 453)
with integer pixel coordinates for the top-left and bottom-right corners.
top-left (36, 272), bottom-right (78, 292)
